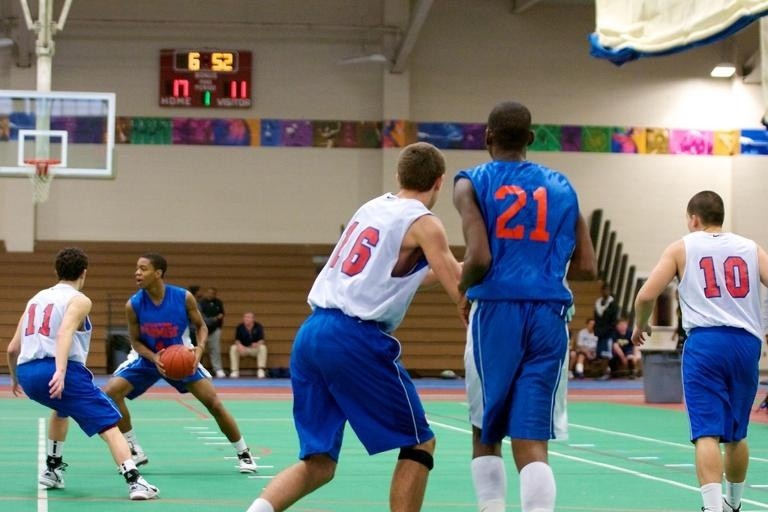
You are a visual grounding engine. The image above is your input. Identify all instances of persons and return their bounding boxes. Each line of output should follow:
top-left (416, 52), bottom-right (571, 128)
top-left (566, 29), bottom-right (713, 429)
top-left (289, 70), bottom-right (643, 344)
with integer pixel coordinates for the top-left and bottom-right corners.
top-left (195, 287), bottom-right (228, 380)
top-left (567, 325), bottom-right (576, 381)
top-left (576, 318), bottom-right (611, 380)
top-left (186, 281), bottom-right (200, 347)
top-left (247, 141), bottom-right (476, 512)
top-left (631, 190), bottom-right (767, 511)
top-left (594, 286), bottom-right (618, 377)
top-left (103, 255), bottom-right (260, 474)
top-left (600, 319), bottom-right (636, 380)
top-left (231, 311), bottom-right (268, 380)
top-left (7, 246), bottom-right (160, 500)
top-left (454, 100), bottom-right (597, 512)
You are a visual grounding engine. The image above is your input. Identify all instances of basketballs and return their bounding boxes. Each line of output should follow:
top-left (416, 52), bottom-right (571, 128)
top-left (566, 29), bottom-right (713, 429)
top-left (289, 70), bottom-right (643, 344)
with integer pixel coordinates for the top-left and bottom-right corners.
top-left (160, 345), bottom-right (195, 378)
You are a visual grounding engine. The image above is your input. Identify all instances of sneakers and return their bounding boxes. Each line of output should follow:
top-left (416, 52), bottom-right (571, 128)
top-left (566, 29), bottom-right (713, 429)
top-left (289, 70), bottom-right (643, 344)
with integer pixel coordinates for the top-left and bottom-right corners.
top-left (575, 371), bottom-right (584, 379)
top-left (628, 370), bottom-right (635, 379)
top-left (216, 370), bottom-right (226, 378)
top-left (229, 371), bottom-right (240, 378)
top-left (257, 369), bottom-right (265, 378)
top-left (599, 374), bottom-right (610, 381)
top-left (117, 448), bottom-right (148, 474)
top-left (38, 462), bottom-right (69, 488)
top-left (568, 371), bottom-right (574, 378)
top-left (237, 447), bottom-right (257, 473)
top-left (722, 498), bottom-right (742, 512)
top-left (128, 475), bottom-right (160, 500)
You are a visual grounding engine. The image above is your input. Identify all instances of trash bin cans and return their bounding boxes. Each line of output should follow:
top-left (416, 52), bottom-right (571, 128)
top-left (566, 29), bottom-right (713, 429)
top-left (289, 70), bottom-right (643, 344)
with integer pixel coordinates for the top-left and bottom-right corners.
top-left (640, 349), bottom-right (684, 402)
top-left (105, 329), bottom-right (130, 374)
